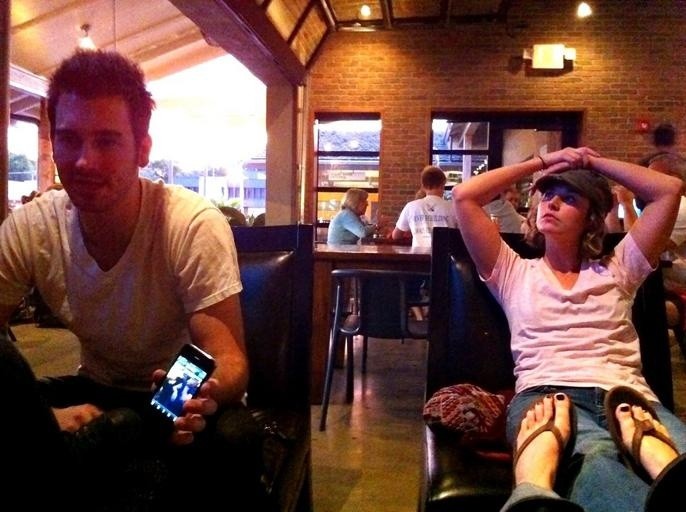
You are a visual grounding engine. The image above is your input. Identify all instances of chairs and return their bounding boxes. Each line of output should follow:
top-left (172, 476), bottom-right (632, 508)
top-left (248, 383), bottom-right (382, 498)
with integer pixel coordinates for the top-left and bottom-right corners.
top-left (319, 269), bottom-right (428, 432)
top-left (417, 226), bottom-right (672, 512)
top-left (232, 223), bottom-right (314, 512)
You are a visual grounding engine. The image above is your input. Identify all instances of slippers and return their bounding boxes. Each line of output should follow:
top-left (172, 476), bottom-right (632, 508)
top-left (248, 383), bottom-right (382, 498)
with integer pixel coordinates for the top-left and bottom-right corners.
top-left (604, 385), bottom-right (681, 486)
top-left (511, 391), bottom-right (575, 490)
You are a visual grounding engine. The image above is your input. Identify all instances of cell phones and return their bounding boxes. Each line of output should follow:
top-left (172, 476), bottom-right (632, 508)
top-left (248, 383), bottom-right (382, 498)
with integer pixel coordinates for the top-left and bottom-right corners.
top-left (150, 343), bottom-right (215, 424)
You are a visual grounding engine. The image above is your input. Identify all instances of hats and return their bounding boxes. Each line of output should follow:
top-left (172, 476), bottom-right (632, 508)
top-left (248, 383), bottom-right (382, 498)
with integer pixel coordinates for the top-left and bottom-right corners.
top-left (535, 167), bottom-right (614, 212)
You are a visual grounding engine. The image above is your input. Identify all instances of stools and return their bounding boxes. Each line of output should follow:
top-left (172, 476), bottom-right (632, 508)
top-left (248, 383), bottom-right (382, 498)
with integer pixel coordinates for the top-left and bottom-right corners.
top-left (5, 325), bottom-right (81, 378)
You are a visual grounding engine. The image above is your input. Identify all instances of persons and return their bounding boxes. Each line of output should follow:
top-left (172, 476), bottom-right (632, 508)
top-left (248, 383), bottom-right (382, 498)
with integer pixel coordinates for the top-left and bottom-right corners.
top-left (0, 48), bottom-right (268, 508)
top-left (327, 189), bottom-right (379, 247)
top-left (156, 374), bottom-right (200, 416)
top-left (390, 164), bottom-right (451, 321)
top-left (451, 143), bottom-right (686, 512)
top-left (601, 124), bottom-right (686, 330)
top-left (505, 384), bottom-right (686, 512)
top-left (480, 187), bottom-right (527, 234)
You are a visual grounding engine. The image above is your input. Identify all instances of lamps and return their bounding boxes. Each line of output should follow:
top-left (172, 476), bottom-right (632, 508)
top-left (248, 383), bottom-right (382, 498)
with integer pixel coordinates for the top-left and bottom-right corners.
top-left (523, 44), bottom-right (576, 69)
top-left (81, 24), bottom-right (96, 49)
